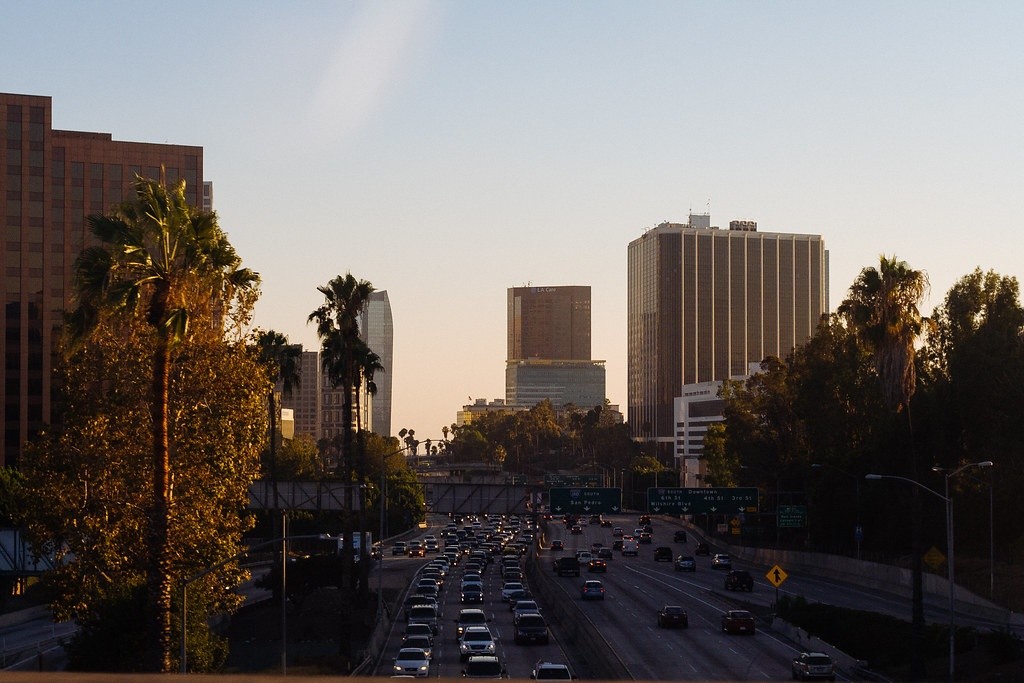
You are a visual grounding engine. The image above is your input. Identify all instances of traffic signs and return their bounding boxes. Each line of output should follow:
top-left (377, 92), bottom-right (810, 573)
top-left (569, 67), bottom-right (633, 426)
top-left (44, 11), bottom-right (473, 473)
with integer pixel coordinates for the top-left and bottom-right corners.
top-left (646, 487), bottom-right (760, 516)
top-left (549, 486), bottom-right (622, 516)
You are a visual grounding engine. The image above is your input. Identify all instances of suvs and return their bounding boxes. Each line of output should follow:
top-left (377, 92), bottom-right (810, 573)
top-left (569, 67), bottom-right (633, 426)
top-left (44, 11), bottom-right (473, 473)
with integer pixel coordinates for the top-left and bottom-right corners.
top-left (458, 625), bottom-right (499, 662)
top-left (460, 654), bottom-right (507, 680)
top-left (514, 613), bottom-right (549, 646)
top-left (791, 651), bottom-right (833, 683)
top-left (512, 600), bottom-right (542, 624)
top-left (710, 553), bottom-right (732, 570)
top-left (556, 557), bottom-right (581, 577)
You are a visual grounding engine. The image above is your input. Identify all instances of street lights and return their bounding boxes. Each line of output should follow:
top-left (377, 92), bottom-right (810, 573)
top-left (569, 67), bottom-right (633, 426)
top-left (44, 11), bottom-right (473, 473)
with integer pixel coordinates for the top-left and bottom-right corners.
top-left (180, 532), bottom-right (344, 673)
top-left (741, 466), bottom-right (780, 550)
top-left (810, 462), bottom-right (861, 560)
top-left (944, 457), bottom-right (996, 576)
top-left (931, 467), bottom-right (994, 602)
top-left (379, 437), bottom-right (452, 617)
top-left (281, 483), bottom-right (375, 677)
top-left (864, 474), bottom-right (954, 683)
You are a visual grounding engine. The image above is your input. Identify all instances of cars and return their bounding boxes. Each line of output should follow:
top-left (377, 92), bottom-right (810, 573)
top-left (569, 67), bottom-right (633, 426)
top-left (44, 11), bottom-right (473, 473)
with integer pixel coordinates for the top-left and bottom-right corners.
top-left (460, 573), bottom-right (484, 593)
top-left (588, 558), bottom-right (607, 573)
top-left (500, 583), bottom-right (528, 603)
top-left (673, 530), bottom-right (687, 544)
top-left (721, 610), bottom-right (755, 636)
top-left (657, 605), bottom-right (688, 629)
top-left (653, 546), bottom-right (673, 562)
top-left (530, 661), bottom-right (577, 681)
top-left (507, 590), bottom-right (537, 613)
top-left (391, 647), bottom-right (432, 678)
top-left (369, 513), bottom-right (655, 664)
top-left (580, 579), bottom-right (605, 602)
top-left (674, 555), bottom-right (696, 572)
top-left (725, 570), bottom-right (754, 591)
top-left (460, 583), bottom-right (484, 605)
